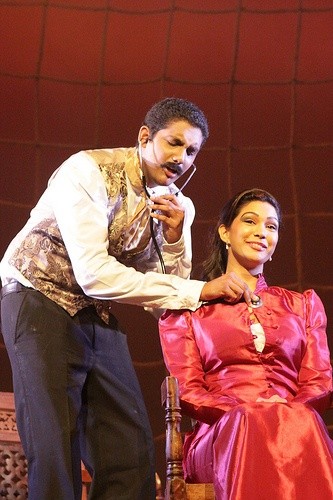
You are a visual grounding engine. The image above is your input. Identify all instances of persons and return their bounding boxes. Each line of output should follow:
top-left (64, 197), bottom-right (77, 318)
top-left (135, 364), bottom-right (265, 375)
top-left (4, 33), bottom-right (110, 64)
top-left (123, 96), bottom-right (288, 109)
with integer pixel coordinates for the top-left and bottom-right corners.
top-left (156, 186), bottom-right (333, 500)
top-left (0, 99), bottom-right (264, 500)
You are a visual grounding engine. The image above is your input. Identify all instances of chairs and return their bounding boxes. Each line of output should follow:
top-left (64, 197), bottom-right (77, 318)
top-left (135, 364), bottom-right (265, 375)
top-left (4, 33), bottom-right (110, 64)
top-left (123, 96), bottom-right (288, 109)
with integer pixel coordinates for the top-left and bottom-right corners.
top-left (160, 376), bottom-right (215, 499)
top-left (1, 392), bottom-right (87, 499)
